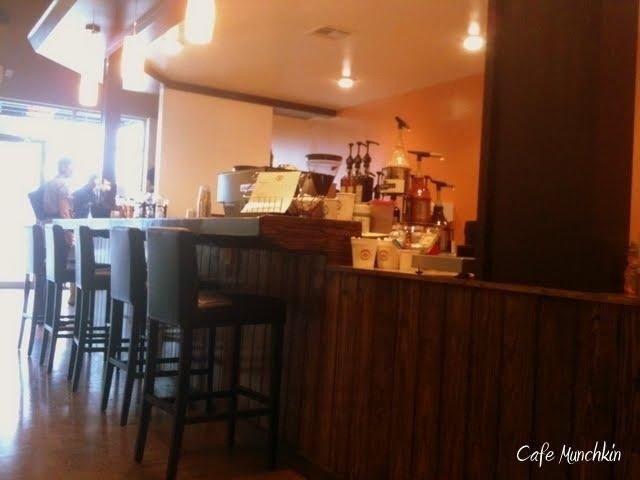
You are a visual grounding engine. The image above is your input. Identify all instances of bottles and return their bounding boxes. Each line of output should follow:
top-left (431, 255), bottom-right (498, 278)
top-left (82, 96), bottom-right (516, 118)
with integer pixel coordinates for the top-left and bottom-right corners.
top-left (110, 192), bottom-right (168, 219)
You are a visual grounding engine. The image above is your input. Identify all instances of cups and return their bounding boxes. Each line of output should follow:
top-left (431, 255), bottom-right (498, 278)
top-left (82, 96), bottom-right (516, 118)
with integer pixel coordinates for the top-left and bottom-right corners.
top-left (395, 248), bottom-right (413, 273)
top-left (350, 237), bottom-right (378, 271)
top-left (337, 191), bottom-right (354, 220)
top-left (368, 198), bottom-right (394, 234)
top-left (324, 196), bottom-right (340, 220)
top-left (375, 239), bottom-right (400, 271)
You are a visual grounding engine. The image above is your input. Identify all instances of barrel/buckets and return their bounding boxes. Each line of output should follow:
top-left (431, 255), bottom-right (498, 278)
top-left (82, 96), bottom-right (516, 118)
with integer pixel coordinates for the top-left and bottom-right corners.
top-left (381, 165), bottom-right (411, 227)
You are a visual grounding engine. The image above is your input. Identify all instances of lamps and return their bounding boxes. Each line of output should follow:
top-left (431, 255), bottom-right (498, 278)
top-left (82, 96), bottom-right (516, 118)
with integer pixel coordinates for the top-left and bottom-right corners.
top-left (76, 16), bottom-right (147, 107)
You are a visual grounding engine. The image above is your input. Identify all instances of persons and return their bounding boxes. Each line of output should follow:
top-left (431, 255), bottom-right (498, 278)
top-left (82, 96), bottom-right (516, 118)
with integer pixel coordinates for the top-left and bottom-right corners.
top-left (43, 155), bottom-right (75, 289)
top-left (69, 173), bottom-right (100, 306)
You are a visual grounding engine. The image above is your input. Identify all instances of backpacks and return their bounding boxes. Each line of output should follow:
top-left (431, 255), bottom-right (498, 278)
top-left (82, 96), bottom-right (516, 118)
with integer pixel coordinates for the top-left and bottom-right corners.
top-left (30, 182), bottom-right (61, 221)
